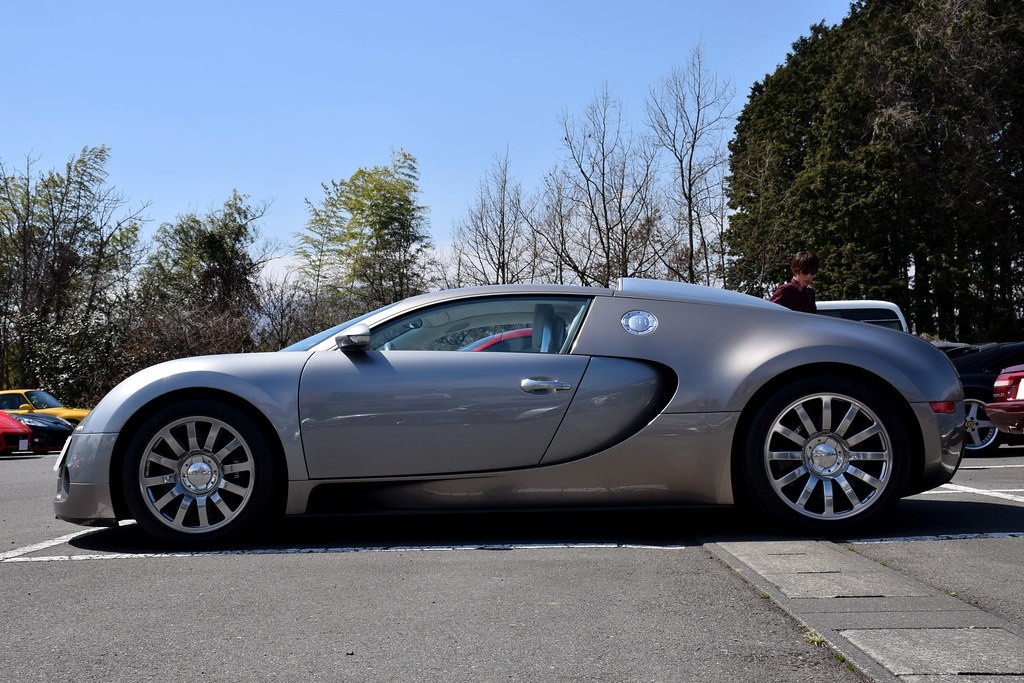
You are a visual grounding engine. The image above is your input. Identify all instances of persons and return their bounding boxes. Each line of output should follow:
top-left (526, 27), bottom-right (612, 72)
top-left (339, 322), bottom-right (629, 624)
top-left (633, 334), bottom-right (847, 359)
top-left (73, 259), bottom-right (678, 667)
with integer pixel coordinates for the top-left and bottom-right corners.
top-left (768, 251), bottom-right (820, 315)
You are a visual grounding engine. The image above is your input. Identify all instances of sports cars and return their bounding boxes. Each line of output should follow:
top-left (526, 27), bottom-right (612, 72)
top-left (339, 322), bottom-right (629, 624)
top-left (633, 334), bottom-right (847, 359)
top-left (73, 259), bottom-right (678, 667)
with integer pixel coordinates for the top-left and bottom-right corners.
top-left (0, 387), bottom-right (92, 455)
top-left (50, 273), bottom-right (965, 541)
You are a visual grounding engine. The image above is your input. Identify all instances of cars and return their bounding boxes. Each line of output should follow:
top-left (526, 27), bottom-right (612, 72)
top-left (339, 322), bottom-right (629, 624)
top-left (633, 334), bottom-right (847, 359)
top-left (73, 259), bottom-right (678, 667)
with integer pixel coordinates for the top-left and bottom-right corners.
top-left (814, 299), bottom-right (910, 335)
top-left (984, 361), bottom-right (1024, 442)
top-left (940, 338), bottom-right (1024, 453)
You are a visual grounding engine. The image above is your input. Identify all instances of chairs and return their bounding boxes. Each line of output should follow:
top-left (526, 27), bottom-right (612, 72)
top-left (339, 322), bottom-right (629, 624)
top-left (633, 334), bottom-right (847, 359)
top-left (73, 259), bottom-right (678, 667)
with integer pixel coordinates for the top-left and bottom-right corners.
top-left (553, 316), bottom-right (567, 353)
top-left (531, 304), bottom-right (555, 353)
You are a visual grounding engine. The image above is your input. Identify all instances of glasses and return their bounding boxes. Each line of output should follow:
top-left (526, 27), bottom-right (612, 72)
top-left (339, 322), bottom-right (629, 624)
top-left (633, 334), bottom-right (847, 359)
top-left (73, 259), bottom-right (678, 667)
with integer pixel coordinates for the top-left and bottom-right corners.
top-left (801, 268), bottom-right (818, 275)
